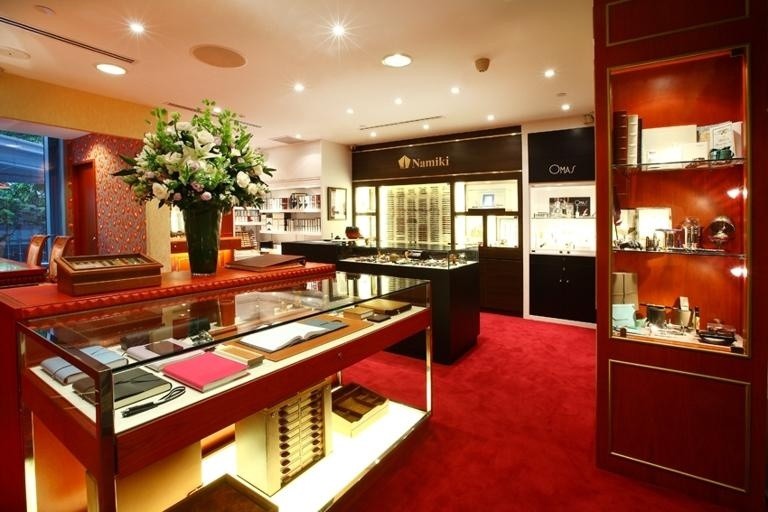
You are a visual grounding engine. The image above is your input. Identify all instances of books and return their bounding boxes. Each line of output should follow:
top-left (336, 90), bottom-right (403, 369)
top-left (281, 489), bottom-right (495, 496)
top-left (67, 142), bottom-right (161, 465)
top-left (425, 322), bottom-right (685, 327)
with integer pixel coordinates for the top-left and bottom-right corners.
top-left (344, 306), bottom-right (375, 321)
top-left (354, 299), bottom-right (411, 315)
top-left (227, 253), bottom-right (306, 272)
top-left (125, 338), bottom-right (250, 394)
top-left (40, 345), bottom-right (172, 410)
top-left (237, 317), bottom-right (349, 353)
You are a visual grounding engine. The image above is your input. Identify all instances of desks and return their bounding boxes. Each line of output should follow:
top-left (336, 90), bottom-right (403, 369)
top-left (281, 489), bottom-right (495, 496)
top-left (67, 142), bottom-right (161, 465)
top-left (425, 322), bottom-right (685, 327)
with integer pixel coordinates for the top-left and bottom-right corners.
top-left (0, 257), bottom-right (48, 287)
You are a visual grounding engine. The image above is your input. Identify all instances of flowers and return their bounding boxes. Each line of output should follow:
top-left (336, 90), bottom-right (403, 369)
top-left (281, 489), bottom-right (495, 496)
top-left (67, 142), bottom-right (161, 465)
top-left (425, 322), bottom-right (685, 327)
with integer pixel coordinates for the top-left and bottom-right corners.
top-left (104, 96), bottom-right (278, 217)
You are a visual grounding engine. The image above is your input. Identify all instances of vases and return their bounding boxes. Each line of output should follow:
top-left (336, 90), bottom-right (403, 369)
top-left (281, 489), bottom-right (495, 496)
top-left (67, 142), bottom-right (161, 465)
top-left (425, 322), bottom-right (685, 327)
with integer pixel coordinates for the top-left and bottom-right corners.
top-left (182, 204), bottom-right (225, 278)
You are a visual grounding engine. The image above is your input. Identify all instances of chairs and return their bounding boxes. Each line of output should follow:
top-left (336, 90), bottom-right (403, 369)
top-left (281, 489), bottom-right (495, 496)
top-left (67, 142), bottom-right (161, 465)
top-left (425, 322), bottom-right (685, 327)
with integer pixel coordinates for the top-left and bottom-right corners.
top-left (26, 233), bottom-right (75, 284)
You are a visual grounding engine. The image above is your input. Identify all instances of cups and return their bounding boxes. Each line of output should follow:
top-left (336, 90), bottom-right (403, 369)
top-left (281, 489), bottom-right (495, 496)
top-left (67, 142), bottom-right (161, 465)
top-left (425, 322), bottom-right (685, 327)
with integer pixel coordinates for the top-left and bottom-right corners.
top-left (646, 305), bottom-right (692, 329)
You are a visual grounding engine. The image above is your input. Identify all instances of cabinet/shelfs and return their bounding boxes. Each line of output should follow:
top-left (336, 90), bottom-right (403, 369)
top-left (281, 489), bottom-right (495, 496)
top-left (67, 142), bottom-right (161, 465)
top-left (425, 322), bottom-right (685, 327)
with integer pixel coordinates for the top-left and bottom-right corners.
top-left (591, 0), bottom-right (767, 511)
top-left (232, 196), bottom-right (322, 249)
